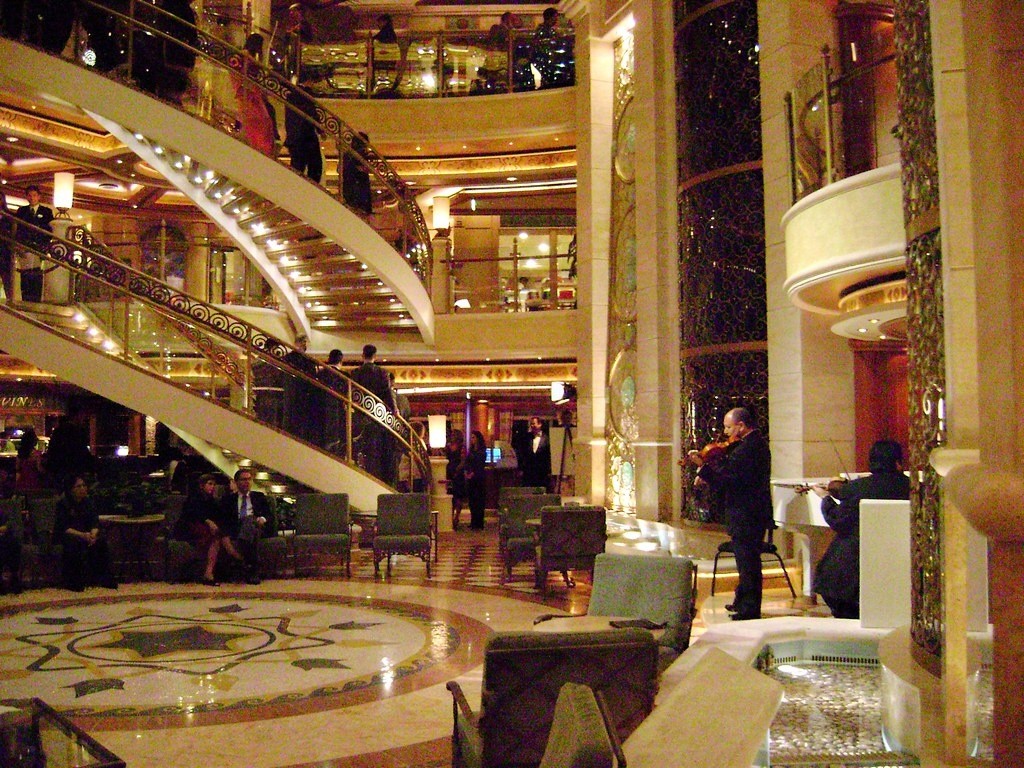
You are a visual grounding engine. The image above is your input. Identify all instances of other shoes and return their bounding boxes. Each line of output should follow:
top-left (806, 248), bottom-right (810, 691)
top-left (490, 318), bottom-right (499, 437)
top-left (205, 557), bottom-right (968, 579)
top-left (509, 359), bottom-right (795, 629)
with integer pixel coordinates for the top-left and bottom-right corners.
top-left (725, 603), bottom-right (738, 611)
top-left (203, 578), bottom-right (220, 586)
top-left (729, 614), bottom-right (742, 621)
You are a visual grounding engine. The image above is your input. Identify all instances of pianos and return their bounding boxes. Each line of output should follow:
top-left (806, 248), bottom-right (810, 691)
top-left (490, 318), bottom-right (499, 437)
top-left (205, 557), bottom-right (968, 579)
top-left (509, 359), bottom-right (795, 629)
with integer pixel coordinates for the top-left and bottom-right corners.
top-left (770, 469), bottom-right (929, 532)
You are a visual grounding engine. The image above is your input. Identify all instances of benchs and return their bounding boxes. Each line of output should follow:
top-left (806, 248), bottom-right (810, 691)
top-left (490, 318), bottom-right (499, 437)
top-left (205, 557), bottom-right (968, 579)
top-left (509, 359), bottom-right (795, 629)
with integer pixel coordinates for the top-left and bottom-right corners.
top-left (166, 495), bottom-right (286, 579)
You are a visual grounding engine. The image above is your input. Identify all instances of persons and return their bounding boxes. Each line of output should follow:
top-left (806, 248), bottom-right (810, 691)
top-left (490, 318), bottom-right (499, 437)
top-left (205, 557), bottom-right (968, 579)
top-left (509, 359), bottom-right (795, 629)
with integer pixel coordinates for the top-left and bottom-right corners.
top-left (0, 418), bottom-right (189, 494)
top-left (0, 509), bottom-right (24, 596)
top-left (445, 428), bottom-right (467, 528)
top-left (0, 0), bottom-right (200, 106)
top-left (688, 407), bottom-right (772, 620)
top-left (0, 185), bottom-right (54, 302)
top-left (174, 469), bottom-right (276, 587)
top-left (462, 431), bottom-right (487, 531)
top-left (518, 417), bottom-right (552, 487)
top-left (372, 13), bottom-right (402, 92)
top-left (210, 12), bottom-right (234, 63)
top-left (814, 439), bottom-right (923, 619)
top-left (486, 8), bottom-right (561, 95)
top-left (252, 333), bottom-right (425, 493)
top-left (51, 472), bottom-right (119, 591)
top-left (343, 132), bottom-right (372, 221)
top-left (231, 33), bottom-right (327, 189)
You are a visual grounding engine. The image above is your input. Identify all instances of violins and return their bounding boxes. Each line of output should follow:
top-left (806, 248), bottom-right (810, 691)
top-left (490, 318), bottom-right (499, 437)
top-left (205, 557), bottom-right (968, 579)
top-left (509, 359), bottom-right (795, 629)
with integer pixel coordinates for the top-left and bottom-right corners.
top-left (793, 477), bottom-right (852, 497)
top-left (677, 435), bottom-right (742, 469)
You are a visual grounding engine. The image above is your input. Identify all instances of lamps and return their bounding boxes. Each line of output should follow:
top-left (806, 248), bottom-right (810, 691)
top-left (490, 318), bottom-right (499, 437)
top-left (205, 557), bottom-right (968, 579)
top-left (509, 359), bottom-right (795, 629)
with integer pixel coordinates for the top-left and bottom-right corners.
top-left (52, 171), bottom-right (74, 222)
top-left (428, 415), bottom-right (446, 456)
top-left (551, 382), bottom-right (578, 406)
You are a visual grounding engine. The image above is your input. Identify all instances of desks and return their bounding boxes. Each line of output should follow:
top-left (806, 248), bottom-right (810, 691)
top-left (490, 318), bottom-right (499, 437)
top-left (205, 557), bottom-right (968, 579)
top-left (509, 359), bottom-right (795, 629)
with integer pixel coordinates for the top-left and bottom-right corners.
top-left (525, 518), bottom-right (578, 590)
top-left (97, 514), bottom-right (165, 581)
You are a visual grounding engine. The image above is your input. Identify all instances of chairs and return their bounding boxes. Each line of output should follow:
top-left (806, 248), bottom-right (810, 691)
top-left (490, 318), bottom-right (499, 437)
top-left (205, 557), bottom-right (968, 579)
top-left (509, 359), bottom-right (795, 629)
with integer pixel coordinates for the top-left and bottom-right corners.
top-left (446, 626), bottom-right (658, 768)
top-left (294, 493), bottom-right (353, 580)
top-left (0, 497), bottom-right (42, 585)
top-left (372, 493), bottom-right (432, 579)
top-left (712, 507), bottom-right (797, 597)
top-left (531, 551), bottom-right (698, 671)
top-left (499, 486), bottom-right (609, 591)
top-left (30, 497), bottom-right (116, 583)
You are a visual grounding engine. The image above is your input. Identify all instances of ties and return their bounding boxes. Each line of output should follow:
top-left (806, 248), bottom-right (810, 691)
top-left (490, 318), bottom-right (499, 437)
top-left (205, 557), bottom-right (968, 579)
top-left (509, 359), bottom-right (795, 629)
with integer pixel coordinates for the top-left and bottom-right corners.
top-left (240, 496), bottom-right (247, 523)
